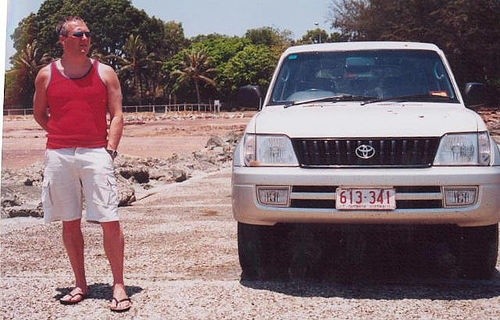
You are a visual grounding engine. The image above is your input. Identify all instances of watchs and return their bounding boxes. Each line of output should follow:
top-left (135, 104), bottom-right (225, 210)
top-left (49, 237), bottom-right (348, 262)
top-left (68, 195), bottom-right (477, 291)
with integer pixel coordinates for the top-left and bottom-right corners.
top-left (107, 149), bottom-right (117, 157)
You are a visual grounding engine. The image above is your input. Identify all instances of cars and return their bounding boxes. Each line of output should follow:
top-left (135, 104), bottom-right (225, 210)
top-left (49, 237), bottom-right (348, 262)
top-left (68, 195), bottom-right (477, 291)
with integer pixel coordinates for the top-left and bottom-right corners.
top-left (230, 42), bottom-right (500, 227)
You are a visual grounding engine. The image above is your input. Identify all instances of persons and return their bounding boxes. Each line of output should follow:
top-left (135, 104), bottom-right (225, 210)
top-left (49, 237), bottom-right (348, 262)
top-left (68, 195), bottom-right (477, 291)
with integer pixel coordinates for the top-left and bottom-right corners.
top-left (33, 16), bottom-right (131, 311)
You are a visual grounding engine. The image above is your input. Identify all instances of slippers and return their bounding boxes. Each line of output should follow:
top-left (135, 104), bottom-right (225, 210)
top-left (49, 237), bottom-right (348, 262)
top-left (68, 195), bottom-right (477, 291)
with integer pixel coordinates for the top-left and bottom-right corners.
top-left (110, 297), bottom-right (130, 311)
top-left (59, 293), bottom-right (83, 304)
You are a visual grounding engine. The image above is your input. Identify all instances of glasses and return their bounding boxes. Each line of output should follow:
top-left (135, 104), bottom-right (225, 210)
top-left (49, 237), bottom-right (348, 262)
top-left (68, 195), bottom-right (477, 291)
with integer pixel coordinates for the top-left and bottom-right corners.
top-left (72, 32), bottom-right (90, 37)
